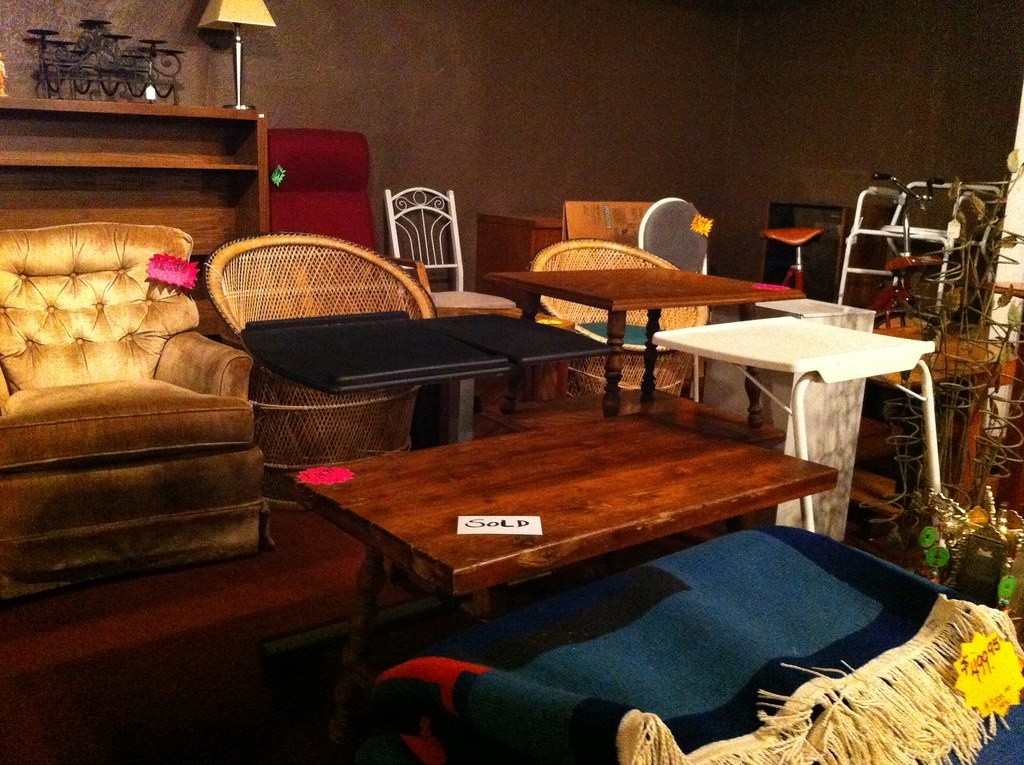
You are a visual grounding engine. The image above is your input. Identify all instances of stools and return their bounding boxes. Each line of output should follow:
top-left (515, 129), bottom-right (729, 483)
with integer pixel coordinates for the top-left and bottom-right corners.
top-left (758, 227), bottom-right (825, 291)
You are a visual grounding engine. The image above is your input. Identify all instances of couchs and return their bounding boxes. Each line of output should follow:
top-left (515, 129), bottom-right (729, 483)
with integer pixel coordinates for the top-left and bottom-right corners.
top-left (0, 220), bottom-right (263, 599)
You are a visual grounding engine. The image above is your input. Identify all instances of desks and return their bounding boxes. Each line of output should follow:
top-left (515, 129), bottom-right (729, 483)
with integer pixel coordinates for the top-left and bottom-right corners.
top-left (650, 316), bottom-right (947, 549)
top-left (867, 324), bottom-right (1018, 515)
top-left (480, 268), bottom-right (806, 430)
top-left (281, 412), bottom-right (840, 747)
top-left (242, 309), bottom-right (616, 586)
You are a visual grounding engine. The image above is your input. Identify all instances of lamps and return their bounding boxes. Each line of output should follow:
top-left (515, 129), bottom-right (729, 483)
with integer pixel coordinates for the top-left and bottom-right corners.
top-left (196, 0), bottom-right (277, 110)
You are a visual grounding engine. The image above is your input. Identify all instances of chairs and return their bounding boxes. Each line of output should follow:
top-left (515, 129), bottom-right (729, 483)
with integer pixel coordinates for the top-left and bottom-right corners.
top-left (266, 128), bottom-right (433, 296)
top-left (527, 239), bottom-right (708, 396)
top-left (384, 186), bottom-right (516, 413)
top-left (204, 232), bottom-right (437, 549)
top-left (638, 198), bottom-right (708, 402)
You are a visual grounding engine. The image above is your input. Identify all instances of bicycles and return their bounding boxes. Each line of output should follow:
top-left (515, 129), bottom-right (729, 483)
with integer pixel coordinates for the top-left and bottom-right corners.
top-left (760, 170), bottom-right (947, 388)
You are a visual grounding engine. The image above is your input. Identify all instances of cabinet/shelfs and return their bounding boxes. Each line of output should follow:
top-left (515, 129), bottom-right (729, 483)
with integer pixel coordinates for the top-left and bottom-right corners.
top-left (477, 213), bottom-right (563, 307)
top-left (0, 97), bottom-right (267, 337)
